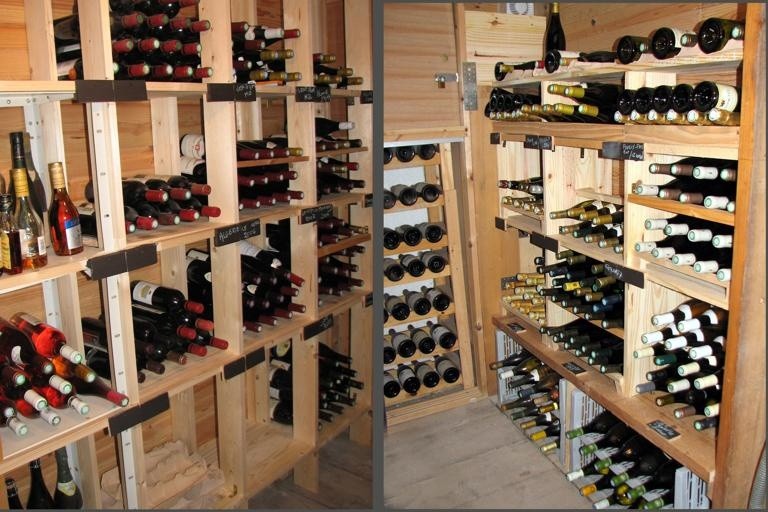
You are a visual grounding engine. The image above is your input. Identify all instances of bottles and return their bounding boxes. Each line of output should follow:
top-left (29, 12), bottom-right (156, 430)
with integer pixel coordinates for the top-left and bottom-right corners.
top-left (0, 115), bottom-right (366, 512)
top-left (3, 3), bottom-right (381, 97)
top-left (483, 1), bottom-right (749, 512)
top-left (382, 143), bottom-right (461, 396)
